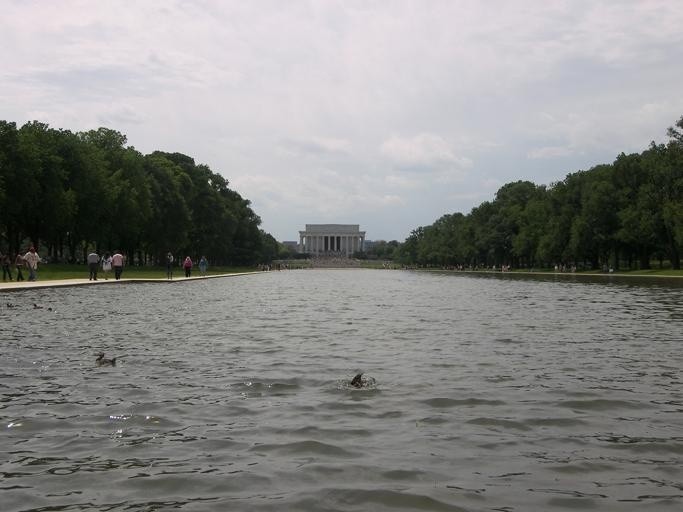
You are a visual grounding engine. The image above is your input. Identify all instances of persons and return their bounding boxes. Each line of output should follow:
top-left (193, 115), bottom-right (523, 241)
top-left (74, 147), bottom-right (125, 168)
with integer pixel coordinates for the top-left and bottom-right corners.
top-left (1, 253), bottom-right (14, 280)
top-left (112, 249), bottom-right (124, 280)
top-left (183, 255), bottom-right (193, 277)
top-left (15, 251), bottom-right (26, 282)
top-left (198, 255), bottom-right (208, 275)
top-left (23, 246), bottom-right (47, 281)
top-left (165, 251), bottom-right (174, 279)
top-left (404, 263), bottom-right (615, 274)
top-left (87, 251), bottom-right (101, 280)
top-left (100, 251), bottom-right (113, 280)
